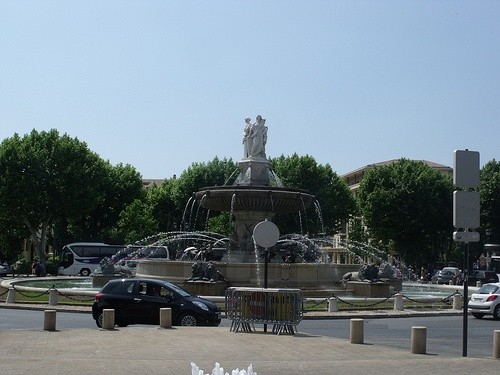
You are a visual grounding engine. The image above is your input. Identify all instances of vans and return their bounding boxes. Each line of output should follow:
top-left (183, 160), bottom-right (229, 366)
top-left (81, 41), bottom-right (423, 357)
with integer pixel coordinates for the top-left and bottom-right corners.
top-left (444, 268), bottom-right (462, 284)
top-left (92, 279), bottom-right (221, 328)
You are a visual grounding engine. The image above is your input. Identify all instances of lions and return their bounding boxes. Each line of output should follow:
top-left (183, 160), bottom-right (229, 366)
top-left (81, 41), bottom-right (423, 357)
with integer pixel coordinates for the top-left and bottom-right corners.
top-left (184, 262), bottom-right (225, 282)
top-left (342, 263), bottom-right (386, 283)
top-left (98, 257), bottom-right (132, 275)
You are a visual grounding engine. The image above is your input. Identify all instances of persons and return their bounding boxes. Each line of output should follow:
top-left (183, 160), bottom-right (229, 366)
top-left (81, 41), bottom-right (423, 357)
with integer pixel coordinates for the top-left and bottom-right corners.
top-left (262, 118), bottom-right (268, 157)
top-left (242, 117), bottom-right (252, 159)
top-left (3, 259), bottom-right (41, 274)
top-left (396, 264), bottom-right (469, 286)
top-left (251, 115), bottom-right (265, 156)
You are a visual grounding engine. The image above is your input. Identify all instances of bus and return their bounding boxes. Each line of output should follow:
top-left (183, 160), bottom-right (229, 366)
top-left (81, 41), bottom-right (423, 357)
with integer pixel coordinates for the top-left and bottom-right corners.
top-left (57, 243), bottom-right (170, 276)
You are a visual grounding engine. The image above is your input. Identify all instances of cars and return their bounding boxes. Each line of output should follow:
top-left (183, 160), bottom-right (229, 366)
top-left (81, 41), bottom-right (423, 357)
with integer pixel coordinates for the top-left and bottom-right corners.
top-left (464, 271), bottom-right (499, 287)
top-left (468, 283), bottom-right (500, 320)
top-left (430, 271), bottom-right (457, 285)
